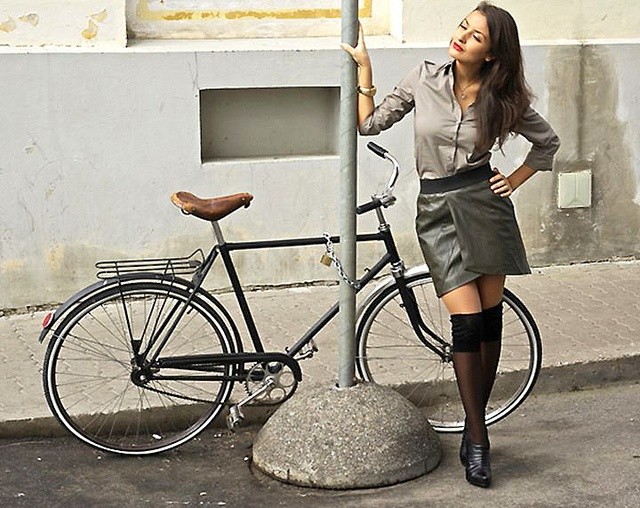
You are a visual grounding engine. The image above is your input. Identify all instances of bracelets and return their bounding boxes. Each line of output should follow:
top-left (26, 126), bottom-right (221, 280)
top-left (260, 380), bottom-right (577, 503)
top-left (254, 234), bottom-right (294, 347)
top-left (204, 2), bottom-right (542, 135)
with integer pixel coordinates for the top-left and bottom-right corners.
top-left (353, 82), bottom-right (377, 99)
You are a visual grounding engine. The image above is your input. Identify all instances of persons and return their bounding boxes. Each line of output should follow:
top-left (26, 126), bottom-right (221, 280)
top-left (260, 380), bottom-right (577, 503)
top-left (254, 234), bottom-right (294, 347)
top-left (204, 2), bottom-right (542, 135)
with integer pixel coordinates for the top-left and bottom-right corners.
top-left (338, 2), bottom-right (557, 487)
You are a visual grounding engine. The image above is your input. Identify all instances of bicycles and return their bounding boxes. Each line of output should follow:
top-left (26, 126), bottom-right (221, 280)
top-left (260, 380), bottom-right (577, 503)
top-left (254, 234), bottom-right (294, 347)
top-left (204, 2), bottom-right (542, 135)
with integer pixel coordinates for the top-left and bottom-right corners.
top-left (39, 142), bottom-right (542, 456)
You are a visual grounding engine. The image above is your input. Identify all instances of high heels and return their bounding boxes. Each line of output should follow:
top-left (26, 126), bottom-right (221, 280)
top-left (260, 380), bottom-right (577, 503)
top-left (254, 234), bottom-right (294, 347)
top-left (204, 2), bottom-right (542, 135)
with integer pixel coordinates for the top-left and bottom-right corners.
top-left (459, 418), bottom-right (469, 466)
top-left (467, 439), bottom-right (491, 488)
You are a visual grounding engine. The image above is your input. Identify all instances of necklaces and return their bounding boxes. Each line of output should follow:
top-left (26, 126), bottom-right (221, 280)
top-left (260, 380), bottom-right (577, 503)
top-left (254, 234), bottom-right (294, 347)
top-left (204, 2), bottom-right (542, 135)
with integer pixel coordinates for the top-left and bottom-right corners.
top-left (454, 77), bottom-right (479, 100)
